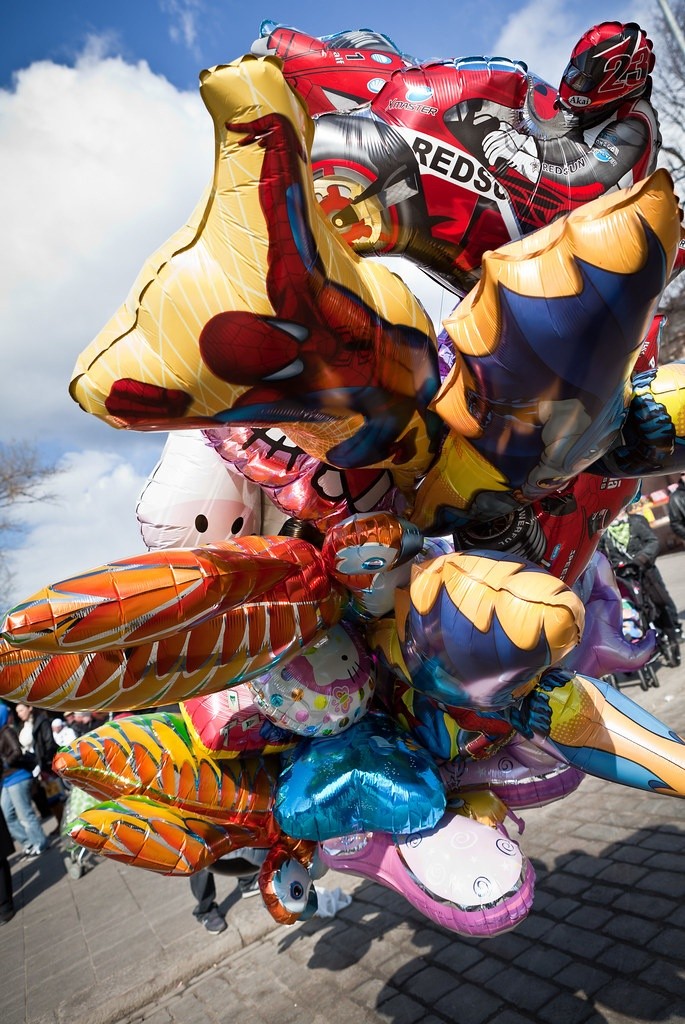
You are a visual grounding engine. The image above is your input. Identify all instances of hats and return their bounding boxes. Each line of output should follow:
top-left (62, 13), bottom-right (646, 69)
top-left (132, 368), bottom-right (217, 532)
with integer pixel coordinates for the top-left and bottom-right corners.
top-left (82, 712), bottom-right (91, 716)
top-left (52, 719), bottom-right (62, 727)
top-left (64, 711), bottom-right (73, 717)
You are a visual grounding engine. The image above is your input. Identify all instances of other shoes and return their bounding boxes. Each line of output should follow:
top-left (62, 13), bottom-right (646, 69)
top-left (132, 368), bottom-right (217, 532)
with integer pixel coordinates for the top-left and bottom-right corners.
top-left (24, 839), bottom-right (50, 855)
top-left (1, 904), bottom-right (15, 922)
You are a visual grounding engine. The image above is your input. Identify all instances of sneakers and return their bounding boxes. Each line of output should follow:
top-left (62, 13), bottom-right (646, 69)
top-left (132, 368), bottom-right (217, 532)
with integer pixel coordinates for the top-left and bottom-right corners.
top-left (238, 879), bottom-right (260, 899)
top-left (195, 902), bottom-right (227, 935)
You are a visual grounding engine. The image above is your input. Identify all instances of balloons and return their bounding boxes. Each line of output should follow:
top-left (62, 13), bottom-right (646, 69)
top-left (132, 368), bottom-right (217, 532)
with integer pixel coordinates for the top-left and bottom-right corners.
top-left (0, 14), bottom-right (685, 939)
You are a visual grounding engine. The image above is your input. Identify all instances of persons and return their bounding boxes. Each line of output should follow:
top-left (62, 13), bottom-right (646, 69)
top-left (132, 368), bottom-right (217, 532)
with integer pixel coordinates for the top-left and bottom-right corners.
top-left (0, 703), bottom-right (134, 926)
top-left (597, 506), bottom-right (685, 644)
top-left (190, 846), bottom-right (269, 936)
top-left (667, 472), bottom-right (685, 540)
